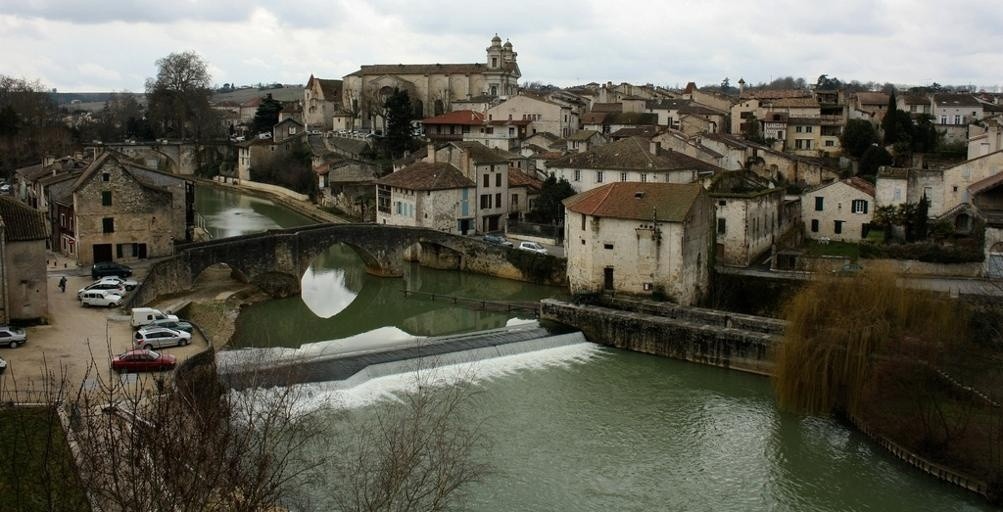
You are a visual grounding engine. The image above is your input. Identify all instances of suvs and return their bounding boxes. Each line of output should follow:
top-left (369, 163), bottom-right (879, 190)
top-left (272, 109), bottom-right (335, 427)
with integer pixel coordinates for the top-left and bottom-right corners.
top-left (483, 233), bottom-right (513, 249)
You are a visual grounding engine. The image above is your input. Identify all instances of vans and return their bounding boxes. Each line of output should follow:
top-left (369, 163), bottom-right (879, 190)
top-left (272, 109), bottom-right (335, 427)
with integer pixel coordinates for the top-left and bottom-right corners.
top-left (259, 133), bottom-right (272, 140)
top-left (132, 308), bottom-right (192, 349)
top-left (518, 241), bottom-right (548, 255)
top-left (91, 262), bottom-right (132, 281)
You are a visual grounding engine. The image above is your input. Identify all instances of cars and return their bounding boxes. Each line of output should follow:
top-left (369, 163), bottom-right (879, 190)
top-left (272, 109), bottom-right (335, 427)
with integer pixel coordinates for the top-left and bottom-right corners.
top-left (229, 136), bottom-right (244, 143)
top-left (0, 182), bottom-right (11, 192)
top-left (112, 351), bottom-right (176, 374)
top-left (76, 274), bottom-right (137, 309)
top-left (312, 129), bottom-right (375, 138)
top-left (0, 325), bottom-right (27, 349)
top-left (0, 356), bottom-right (7, 375)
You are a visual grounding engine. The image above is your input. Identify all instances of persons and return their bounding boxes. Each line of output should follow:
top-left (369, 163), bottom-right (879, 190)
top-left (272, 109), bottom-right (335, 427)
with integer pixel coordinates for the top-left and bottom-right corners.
top-left (59, 276), bottom-right (67, 292)
